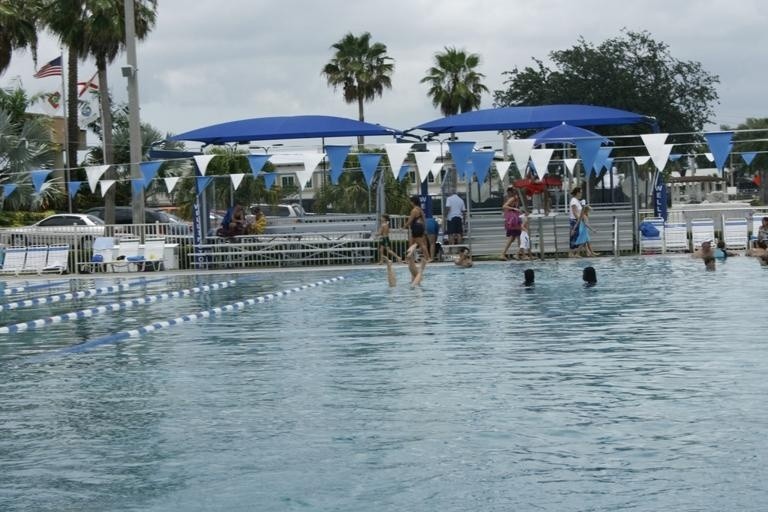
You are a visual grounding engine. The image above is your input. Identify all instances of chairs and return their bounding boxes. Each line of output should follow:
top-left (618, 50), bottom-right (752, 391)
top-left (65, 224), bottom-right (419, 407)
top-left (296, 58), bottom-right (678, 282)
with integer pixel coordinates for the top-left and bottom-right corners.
top-left (639, 213), bottom-right (768, 254)
top-left (78, 236), bottom-right (166, 274)
top-left (0, 242), bottom-right (69, 274)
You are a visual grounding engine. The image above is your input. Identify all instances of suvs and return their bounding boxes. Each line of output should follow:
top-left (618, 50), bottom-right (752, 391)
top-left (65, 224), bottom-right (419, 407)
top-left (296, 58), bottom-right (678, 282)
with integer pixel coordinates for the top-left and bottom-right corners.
top-left (249, 203), bottom-right (309, 220)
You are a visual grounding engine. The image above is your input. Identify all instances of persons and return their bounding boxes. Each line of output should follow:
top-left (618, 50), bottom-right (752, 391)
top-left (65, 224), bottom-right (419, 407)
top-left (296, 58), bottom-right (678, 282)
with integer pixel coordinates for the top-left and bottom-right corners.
top-left (217, 199), bottom-right (267, 244)
top-left (523, 269), bottom-right (535, 286)
top-left (499, 187), bottom-right (603, 261)
top-left (376, 188), bottom-right (474, 288)
top-left (693, 217), bottom-right (768, 272)
top-left (582, 266), bottom-right (597, 288)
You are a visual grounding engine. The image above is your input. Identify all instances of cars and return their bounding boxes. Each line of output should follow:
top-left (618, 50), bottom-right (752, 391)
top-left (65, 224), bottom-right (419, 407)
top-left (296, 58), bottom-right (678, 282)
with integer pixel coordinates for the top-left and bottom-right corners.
top-left (2, 212), bottom-right (125, 249)
top-left (84, 206), bottom-right (194, 233)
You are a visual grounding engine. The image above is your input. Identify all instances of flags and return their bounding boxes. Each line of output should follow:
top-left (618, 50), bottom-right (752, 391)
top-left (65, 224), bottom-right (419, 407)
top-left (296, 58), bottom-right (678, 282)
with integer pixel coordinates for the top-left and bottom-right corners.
top-left (32, 56), bottom-right (61, 78)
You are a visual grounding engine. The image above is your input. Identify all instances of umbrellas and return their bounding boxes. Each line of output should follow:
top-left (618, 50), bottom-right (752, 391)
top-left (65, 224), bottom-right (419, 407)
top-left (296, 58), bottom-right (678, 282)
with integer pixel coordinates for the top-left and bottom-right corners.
top-left (524, 121), bottom-right (614, 213)
